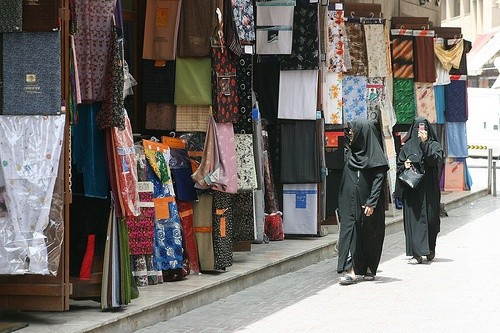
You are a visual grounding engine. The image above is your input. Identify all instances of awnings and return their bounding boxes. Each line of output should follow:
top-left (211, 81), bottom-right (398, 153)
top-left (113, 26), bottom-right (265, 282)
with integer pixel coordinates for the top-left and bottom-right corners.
top-left (466, 29), bottom-right (500, 76)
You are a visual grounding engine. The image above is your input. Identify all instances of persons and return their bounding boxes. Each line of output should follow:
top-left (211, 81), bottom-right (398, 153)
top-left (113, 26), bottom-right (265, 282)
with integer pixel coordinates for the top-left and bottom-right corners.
top-left (396, 116), bottom-right (445, 264)
top-left (337, 117), bottom-right (390, 284)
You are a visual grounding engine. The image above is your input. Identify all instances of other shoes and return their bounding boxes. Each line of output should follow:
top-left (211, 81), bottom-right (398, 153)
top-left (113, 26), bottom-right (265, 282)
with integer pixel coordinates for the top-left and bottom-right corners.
top-left (408, 256), bottom-right (423, 264)
top-left (363, 272), bottom-right (374, 280)
top-left (338, 274), bottom-right (357, 285)
top-left (427, 255), bottom-right (434, 260)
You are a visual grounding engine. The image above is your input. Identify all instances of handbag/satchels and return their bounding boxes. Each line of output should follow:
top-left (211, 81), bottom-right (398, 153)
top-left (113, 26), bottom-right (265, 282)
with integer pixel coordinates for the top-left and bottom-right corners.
top-left (399, 163), bottom-right (425, 192)
